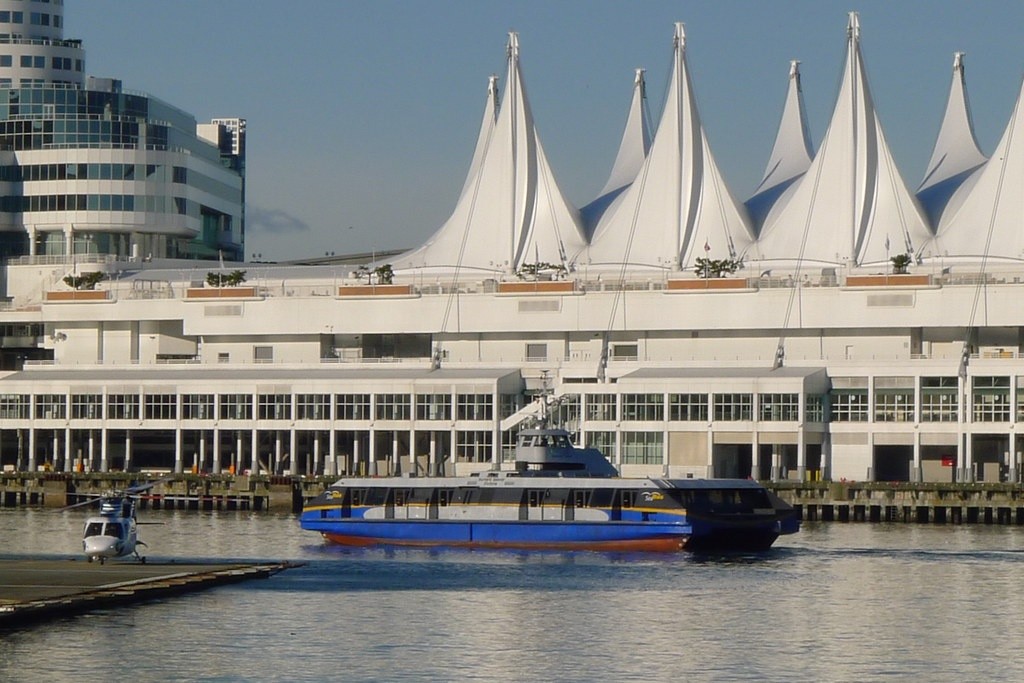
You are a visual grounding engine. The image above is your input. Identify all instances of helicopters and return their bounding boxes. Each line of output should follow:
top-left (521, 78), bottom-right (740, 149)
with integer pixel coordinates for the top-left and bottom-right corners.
top-left (47, 472), bottom-right (181, 568)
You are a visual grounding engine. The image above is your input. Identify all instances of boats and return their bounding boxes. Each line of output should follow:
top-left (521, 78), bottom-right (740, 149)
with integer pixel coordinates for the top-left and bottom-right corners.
top-left (297, 368), bottom-right (804, 561)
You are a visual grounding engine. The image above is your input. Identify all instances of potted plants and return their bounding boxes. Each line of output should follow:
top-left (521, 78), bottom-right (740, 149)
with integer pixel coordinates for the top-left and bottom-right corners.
top-left (47, 271), bottom-right (109, 300)
top-left (667, 257), bottom-right (748, 289)
top-left (187, 268), bottom-right (256, 296)
top-left (339, 264), bottom-right (411, 295)
top-left (498, 263), bottom-right (576, 292)
top-left (846, 252), bottom-right (929, 286)
